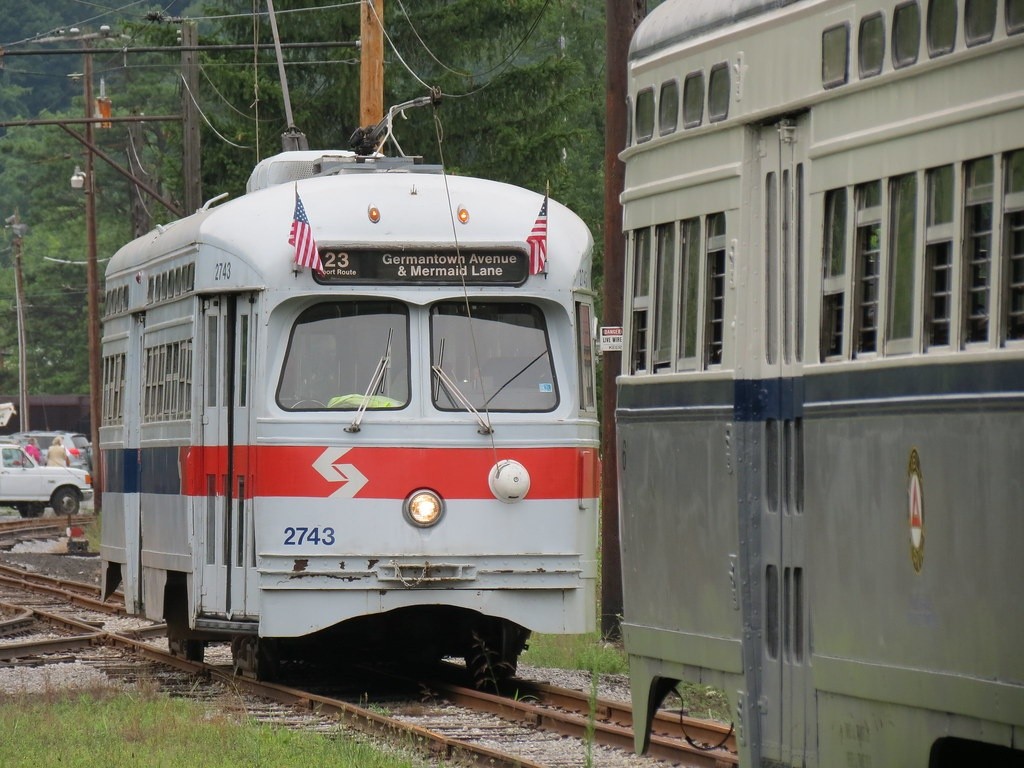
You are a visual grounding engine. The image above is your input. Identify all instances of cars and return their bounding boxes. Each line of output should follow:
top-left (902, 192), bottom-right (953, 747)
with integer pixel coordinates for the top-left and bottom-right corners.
top-left (0, 426), bottom-right (92, 518)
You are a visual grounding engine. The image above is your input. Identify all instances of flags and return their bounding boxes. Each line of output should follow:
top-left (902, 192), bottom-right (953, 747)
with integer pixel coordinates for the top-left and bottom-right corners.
top-left (288, 184), bottom-right (326, 279)
top-left (526, 192), bottom-right (547, 275)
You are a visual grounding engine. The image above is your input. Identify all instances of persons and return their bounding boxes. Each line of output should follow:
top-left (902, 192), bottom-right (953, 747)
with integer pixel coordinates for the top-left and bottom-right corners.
top-left (22, 437), bottom-right (42, 469)
top-left (44, 437), bottom-right (67, 468)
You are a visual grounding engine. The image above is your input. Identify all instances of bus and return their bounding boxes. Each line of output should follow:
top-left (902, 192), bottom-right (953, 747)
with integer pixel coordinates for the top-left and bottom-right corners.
top-left (84, 3), bottom-right (606, 693)
top-left (620, 0), bottom-right (1024, 767)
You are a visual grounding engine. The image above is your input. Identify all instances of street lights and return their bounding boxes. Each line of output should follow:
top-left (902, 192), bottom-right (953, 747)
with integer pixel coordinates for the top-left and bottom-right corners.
top-left (70, 165), bottom-right (102, 516)
top-left (3, 204), bottom-right (31, 434)
